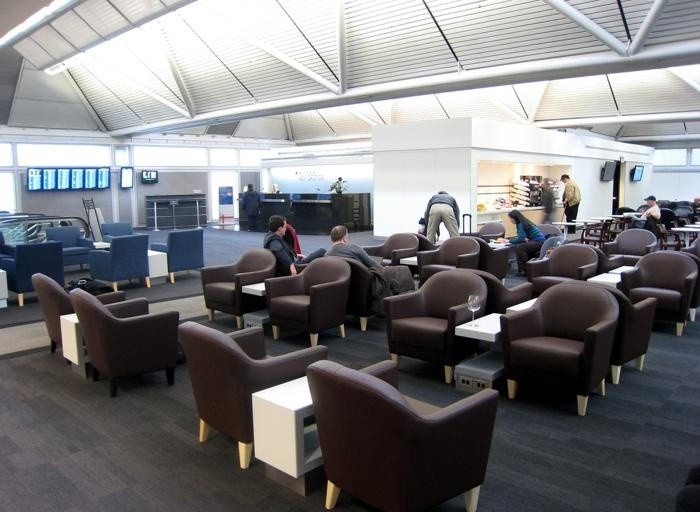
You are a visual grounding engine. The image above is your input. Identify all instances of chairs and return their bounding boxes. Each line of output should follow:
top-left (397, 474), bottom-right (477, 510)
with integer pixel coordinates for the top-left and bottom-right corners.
top-left (69, 287), bottom-right (180, 397)
top-left (88, 233), bottom-right (151, 294)
top-left (101, 222), bottom-right (138, 244)
top-left (306, 358), bottom-right (499, 512)
top-left (621, 250), bottom-right (697, 337)
top-left (265, 256), bottom-right (351, 347)
top-left (270, 255), bottom-right (309, 278)
top-left (357, 200), bottom-right (700, 295)
top-left (201, 248), bottom-right (278, 330)
top-left (150, 227), bottom-right (205, 283)
top-left (31, 273), bottom-right (126, 370)
top-left (46, 223), bottom-right (95, 272)
top-left (384, 269), bottom-right (487, 386)
top-left (1, 240), bottom-right (64, 307)
top-left (500, 282), bottom-right (619, 418)
top-left (461, 268), bottom-right (534, 316)
top-left (582, 283), bottom-right (657, 384)
top-left (325, 255), bottom-right (412, 330)
top-left (179, 322), bottom-right (328, 468)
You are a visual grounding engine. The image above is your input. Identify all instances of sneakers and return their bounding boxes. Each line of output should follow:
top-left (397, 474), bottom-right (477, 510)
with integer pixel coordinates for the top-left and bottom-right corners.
top-left (515, 272), bottom-right (525, 276)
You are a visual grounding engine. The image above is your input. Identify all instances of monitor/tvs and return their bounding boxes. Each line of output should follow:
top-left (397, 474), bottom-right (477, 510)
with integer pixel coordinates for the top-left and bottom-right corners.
top-left (142, 170), bottom-right (158, 184)
top-left (602, 161), bottom-right (617, 182)
top-left (630, 165), bottom-right (643, 182)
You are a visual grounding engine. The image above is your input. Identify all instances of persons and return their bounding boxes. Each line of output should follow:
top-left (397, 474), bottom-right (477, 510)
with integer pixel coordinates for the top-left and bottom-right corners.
top-left (688, 197), bottom-right (700, 224)
top-left (262, 216), bottom-right (326, 276)
top-left (559, 174), bottom-right (580, 234)
top-left (241, 184), bottom-right (263, 231)
top-left (538, 178), bottom-right (557, 224)
top-left (500, 209), bottom-right (546, 276)
top-left (425, 191), bottom-right (460, 249)
top-left (628, 195), bottom-right (661, 229)
top-left (271, 184), bottom-right (282, 194)
top-left (322, 226), bottom-right (380, 269)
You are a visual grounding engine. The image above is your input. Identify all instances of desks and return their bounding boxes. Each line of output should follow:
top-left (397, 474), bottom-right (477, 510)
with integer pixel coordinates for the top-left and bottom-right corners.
top-left (59, 314), bottom-right (92, 379)
top-left (251, 375), bottom-right (324, 496)
top-left (455, 313), bottom-right (503, 394)
top-left (242, 283), bottom-right (272, 334)
top-left (94, 241), bottom-right (110, 251)
top-left (505, 297), bottom-right (538, 313)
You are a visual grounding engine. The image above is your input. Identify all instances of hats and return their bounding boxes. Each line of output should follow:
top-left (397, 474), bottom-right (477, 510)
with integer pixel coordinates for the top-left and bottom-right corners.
top-left (644, 196), bottom-right (656, 201)
top-left (560, 174), bottom-right (569, 182)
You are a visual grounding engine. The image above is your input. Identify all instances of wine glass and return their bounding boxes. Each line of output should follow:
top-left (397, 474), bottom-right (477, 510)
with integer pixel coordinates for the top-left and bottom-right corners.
top-left (467, 294), bottom-right (482, 329)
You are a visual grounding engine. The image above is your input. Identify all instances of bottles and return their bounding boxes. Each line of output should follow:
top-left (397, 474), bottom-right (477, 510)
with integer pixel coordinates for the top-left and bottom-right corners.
top-left (545, 241), bottom-right (560, 257)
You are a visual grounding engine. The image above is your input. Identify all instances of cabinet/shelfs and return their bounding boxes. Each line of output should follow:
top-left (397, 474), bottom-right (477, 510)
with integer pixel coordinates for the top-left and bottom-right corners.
top-left (520, 175), bottom-right (543, 206)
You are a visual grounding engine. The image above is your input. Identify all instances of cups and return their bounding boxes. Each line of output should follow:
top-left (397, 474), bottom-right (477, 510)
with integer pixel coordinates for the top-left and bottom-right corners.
top-left (489, 237), bottom-right (509, 246)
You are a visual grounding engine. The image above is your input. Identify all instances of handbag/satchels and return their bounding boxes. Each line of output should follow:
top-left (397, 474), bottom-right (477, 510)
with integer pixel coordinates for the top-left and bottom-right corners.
top-left (68, 278), bottom-right (114, 295)
